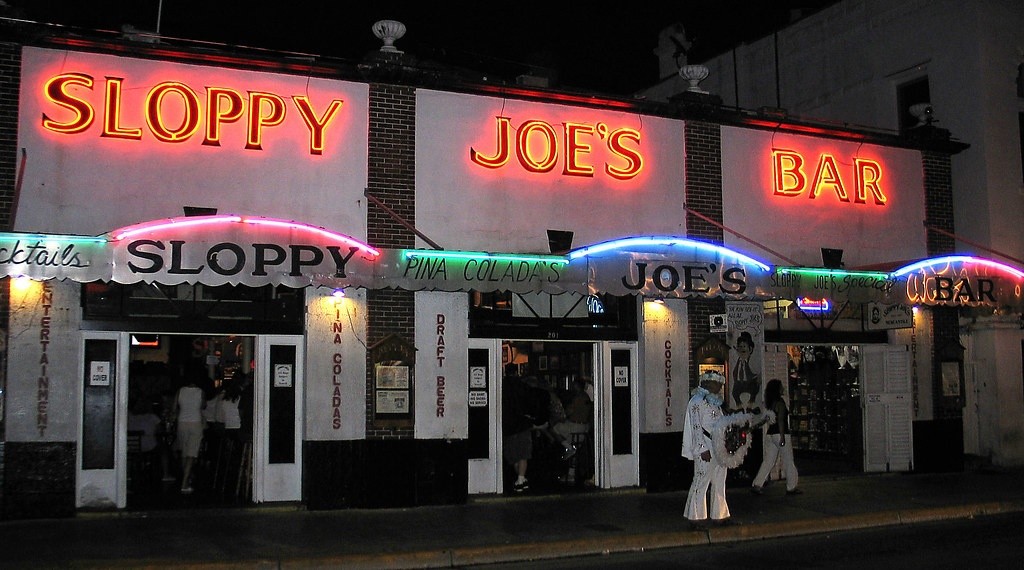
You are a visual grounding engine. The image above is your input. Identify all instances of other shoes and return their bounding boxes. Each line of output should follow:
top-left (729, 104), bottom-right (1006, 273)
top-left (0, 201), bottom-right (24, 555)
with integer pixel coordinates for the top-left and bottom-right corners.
top-left (561, 445), bottom-right (577, 460)
top-left (749, 486), bottom-right (768, 498)
top-left (181, 486), bottom-right (194, 492)
top-left (712, 516), bottom-right (745, 528)
top-left (786, 487), bottom-right (805, 495)
top-left (162, 476), bottom-right (176, 481)
top-left (686, 520), bottom-right (709, 532)
top-left (513, 479), bottom-right (529, 492)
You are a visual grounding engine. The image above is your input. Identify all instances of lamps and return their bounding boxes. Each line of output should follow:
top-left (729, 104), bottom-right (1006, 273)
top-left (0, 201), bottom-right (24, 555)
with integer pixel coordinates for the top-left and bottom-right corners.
top-left (182, 206), bottom-right (218, 217)
top-left (820, 247), bottom-right (845, 270)
top-left (547, 228), bottom-right (574, 254)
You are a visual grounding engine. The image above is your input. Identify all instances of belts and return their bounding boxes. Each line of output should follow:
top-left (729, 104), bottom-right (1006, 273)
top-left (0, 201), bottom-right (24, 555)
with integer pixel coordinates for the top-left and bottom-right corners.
top-left (569, 420), bottom-right (588, 425)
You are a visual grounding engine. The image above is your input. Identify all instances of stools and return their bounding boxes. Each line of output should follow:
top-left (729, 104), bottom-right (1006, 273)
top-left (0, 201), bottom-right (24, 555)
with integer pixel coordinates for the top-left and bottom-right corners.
top-left (213, 429), bottom-right (242, 490)
top-left (571, 432), bottom-right (592, 470)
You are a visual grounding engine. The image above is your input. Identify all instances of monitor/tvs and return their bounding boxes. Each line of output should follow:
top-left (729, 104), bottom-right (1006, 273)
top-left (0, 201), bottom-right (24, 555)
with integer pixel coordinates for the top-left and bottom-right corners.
top-left (132, 334), bottom-right (162, 350)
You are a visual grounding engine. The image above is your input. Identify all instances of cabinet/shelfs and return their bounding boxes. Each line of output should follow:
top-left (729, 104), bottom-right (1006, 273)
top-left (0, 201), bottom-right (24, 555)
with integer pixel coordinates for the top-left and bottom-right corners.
top-left (789, 378), bottom-right (862, 456)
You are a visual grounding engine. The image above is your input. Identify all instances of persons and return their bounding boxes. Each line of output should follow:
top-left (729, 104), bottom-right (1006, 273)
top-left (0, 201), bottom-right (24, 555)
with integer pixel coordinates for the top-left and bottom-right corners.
top-left (501, 363), bottom-right (591, 493)
top-left (750, 379), bottom-right (804, 495)
top-left (123, 360), bottom-right (254, 497)
top-left (681, 370), bottom-right (730, 527)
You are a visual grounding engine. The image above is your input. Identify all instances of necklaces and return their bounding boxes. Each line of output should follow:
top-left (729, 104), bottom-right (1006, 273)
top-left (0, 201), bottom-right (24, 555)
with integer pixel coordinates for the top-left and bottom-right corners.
top-left (691, 387), bottom-right (723, 406)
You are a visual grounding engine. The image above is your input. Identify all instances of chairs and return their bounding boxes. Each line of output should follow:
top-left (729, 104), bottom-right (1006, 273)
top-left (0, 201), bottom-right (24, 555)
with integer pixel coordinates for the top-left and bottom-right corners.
top-left (127, 429), bottom-right (147, 496)
